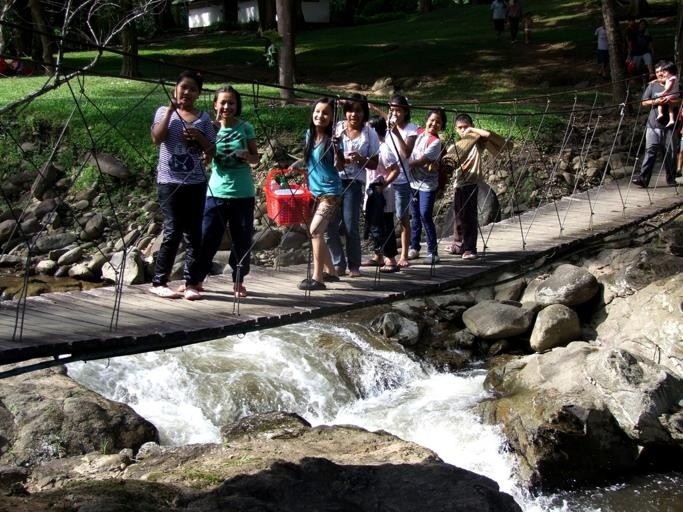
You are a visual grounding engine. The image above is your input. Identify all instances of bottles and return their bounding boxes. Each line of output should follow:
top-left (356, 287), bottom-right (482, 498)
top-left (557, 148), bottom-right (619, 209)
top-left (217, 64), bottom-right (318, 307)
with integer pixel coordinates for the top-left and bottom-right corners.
top-left (278, 177), bottom-right (290, 189)
top-left (268, 178), bottom-right (277, 191)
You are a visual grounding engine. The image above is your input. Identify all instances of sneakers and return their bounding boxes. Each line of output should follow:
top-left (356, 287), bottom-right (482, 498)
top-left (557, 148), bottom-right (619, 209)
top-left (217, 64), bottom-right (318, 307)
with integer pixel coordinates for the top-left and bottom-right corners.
top-left (408, 250), bottom-right (419, 260)
top-left (632, 174), bottom-right (682, 188)
top-left (445, 245), bottom-right (453, 252)
top-left (335, 267), bottom-right (345, 275)
top-left (462, 252), bottom-right (477, 260)
top-left (423, 254), bottom-right (440, 264)
top-left (349, 267), bottom-right (361, 277)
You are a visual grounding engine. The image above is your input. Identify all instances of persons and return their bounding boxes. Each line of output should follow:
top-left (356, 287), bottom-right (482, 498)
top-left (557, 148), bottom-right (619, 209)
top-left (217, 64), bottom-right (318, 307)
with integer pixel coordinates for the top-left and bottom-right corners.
top-left (488, 0), bottom-right (658, 90)
top-left (335, 91), bottom-right (379, 280)
top-left (647, 59), bottom-right (681, 131)
top-left (178, 85), bottom-right (261, 298)
top-left (366, 93), bottom-right (419, 267)
top-left (361, 114), bottom-right (401, 274)
top-left (674, 100), bottom-right (683, 177)
top-left (146, 70), bottom-right (216, 302)
top-left (285, 95), bottom-right (345, 291)
top-left (445, 113), bottom-right (494, 263)
top-left (406, 106), bottom-right (449, 266)
top-left (627, 60), bottom-right (683, 189)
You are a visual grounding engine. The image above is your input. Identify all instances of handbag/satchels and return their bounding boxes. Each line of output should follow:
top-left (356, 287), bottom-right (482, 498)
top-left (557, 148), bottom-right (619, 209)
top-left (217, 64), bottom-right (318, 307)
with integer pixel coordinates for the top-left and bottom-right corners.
top-left (451, 242), bottom-right (464, 255)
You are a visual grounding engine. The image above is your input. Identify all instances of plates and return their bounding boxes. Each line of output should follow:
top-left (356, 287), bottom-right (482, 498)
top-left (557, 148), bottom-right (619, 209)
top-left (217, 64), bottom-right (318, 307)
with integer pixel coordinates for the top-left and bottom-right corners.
top-left (273, 190), bottom-right (305, 195)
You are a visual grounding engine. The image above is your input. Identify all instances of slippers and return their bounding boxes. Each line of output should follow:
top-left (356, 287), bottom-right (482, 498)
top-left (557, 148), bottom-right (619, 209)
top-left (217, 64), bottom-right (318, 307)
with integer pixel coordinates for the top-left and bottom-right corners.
top-left (184, 289), bottom-right (201, 300)
top-left (149, 286), bottom-right (180, 298)
top-left (297, 279), bottom-right (326, 290)
top-left (323, 271), bottom-right (340, 281)
top-left (362, 259), bottom-right (409, 273)
top-left (178, 285), bottom-right (202, 292)
top-left (233, 286), bottom-right (247, 297)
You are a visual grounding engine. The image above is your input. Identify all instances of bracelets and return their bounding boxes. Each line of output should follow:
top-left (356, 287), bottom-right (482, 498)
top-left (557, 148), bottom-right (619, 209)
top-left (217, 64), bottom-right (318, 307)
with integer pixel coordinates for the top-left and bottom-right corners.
top-left (652, 99), bottom-right (655, 105)
